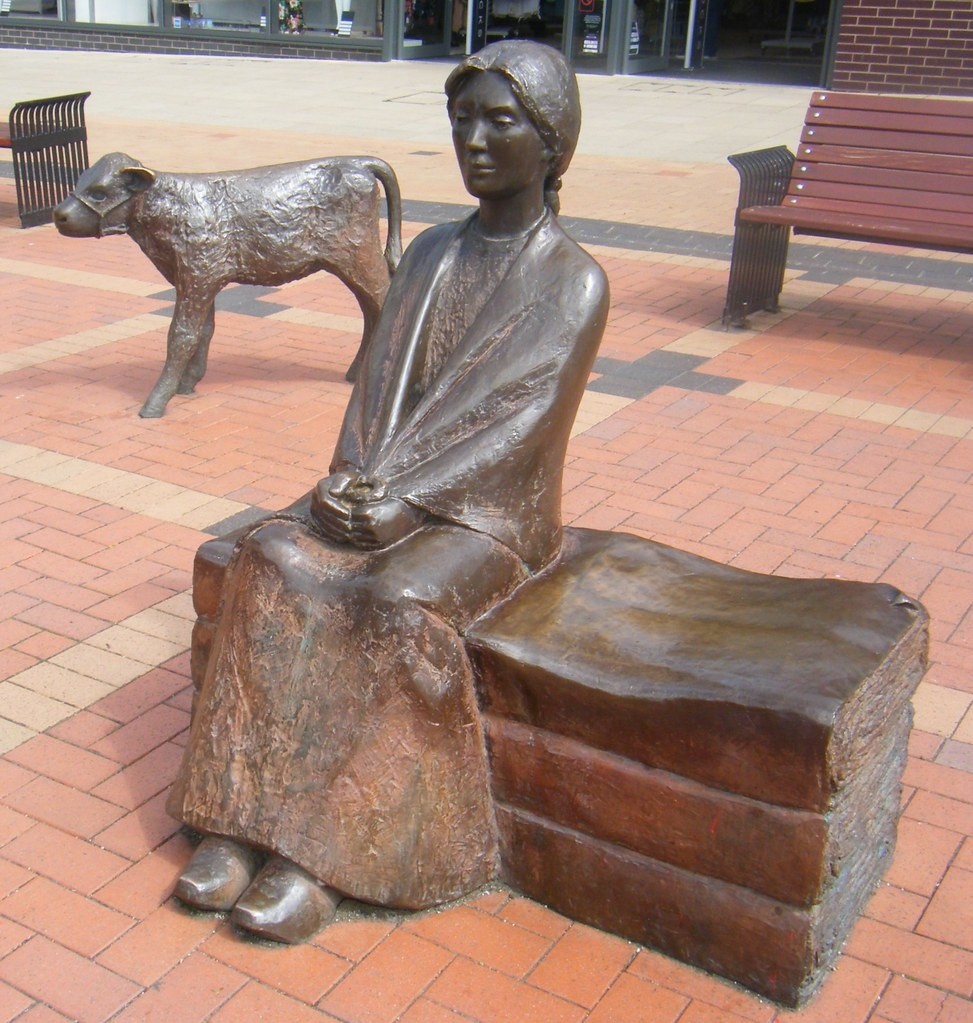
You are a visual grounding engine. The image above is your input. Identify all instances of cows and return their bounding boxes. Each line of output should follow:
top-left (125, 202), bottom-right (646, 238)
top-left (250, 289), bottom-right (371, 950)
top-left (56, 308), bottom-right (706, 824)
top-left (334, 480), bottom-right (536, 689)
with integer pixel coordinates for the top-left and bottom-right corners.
top-left (54, 152), bottom-right (402, 417)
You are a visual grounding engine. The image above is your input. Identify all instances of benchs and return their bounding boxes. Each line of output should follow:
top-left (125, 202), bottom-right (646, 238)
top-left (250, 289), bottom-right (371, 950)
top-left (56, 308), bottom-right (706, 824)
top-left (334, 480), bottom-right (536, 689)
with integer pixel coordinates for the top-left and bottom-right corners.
top-left (721, 90), bottom-right (972, 328)
top-left (1, 90), bottom-right (95, 229)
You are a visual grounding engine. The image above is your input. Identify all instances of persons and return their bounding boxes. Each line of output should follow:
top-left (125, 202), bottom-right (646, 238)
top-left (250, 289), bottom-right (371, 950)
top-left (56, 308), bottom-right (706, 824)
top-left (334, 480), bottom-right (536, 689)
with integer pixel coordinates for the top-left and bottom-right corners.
top-left (164, 39), bottom-right (612, 939)
